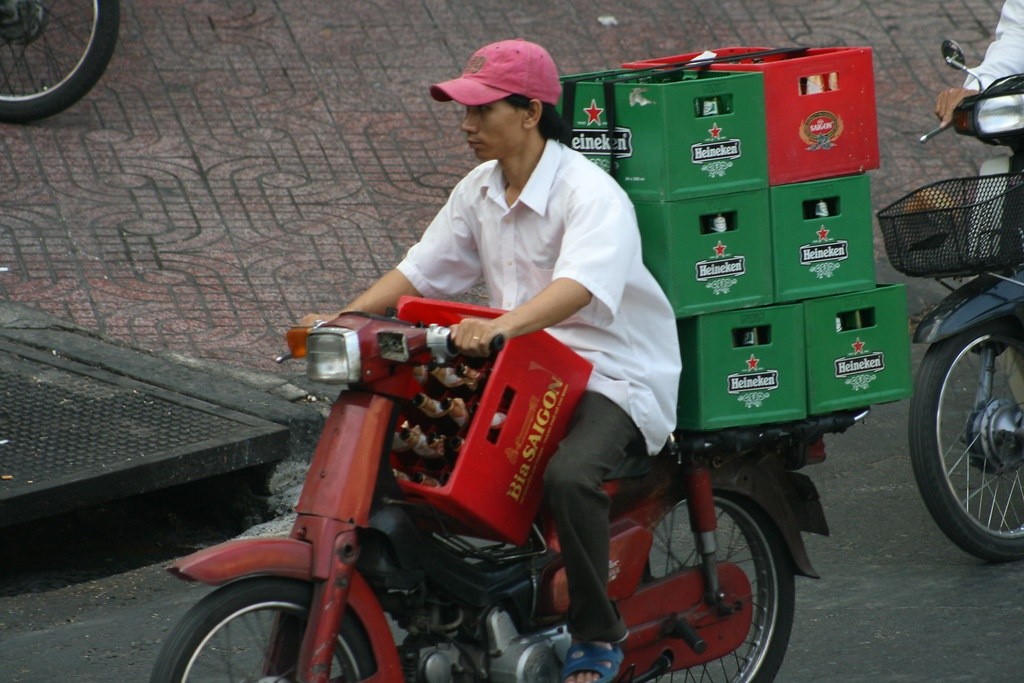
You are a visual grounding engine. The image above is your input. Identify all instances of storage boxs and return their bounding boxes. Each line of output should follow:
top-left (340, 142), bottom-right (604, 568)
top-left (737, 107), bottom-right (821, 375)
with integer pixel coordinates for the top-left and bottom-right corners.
top-left (623, 188), bottom-right (775, 319)
top-left (690, 276), bottom-right (691, 283)
top-left (801, 283), bottom-right (915, 416)
top-left (387, 294), bottom-right (594, 545)
top-left (621, 46), bottom-right (882, 186)
top-left (554, 70), bottom-right (770, 203)
top-left (676, 303), bottom-right (808, 430)
top-left (769, 175), bottom-right (875, 302)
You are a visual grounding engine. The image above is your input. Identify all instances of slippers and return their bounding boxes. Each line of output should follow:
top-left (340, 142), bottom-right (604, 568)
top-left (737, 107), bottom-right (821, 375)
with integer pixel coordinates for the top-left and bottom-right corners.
top-left (562, 643), bottom-right (624, 683)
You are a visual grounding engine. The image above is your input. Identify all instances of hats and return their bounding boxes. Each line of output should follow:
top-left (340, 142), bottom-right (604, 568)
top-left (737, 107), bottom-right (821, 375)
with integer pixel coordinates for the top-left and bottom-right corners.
top-left (430, 38), bottom-right (562, 107)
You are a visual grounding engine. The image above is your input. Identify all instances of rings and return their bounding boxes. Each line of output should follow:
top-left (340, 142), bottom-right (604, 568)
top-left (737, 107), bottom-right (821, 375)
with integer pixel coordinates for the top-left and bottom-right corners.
top-left (473, 337), bottom-right (479, 340)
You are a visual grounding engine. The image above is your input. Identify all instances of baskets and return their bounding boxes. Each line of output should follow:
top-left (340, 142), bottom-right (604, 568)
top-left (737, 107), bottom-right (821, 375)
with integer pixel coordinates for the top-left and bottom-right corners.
top-left (875, 173), bottom-right (1024, 276)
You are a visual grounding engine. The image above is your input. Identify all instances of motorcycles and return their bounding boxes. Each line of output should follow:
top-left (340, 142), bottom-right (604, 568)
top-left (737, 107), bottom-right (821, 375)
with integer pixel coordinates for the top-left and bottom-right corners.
top-left (145, 306), bottom-right (871, 682)
top-left (869, 30), bottom-right (1023, 567)
top-left (0, 0), bottom-right (120, 124)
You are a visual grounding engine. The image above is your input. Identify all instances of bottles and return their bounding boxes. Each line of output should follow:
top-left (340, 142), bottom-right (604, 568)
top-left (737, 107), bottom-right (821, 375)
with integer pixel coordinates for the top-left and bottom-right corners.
top-left (390, 322), bottom-right (508, 490)
top-left (626, 51), bottom-right (841, 116)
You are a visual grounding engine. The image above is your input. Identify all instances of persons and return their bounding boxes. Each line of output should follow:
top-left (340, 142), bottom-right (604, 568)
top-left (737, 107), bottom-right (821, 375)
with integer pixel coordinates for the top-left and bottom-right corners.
top-left (932, 0), bottom-right (1024, 420)
top-left (294, 39), bottom-right (684, 682)
